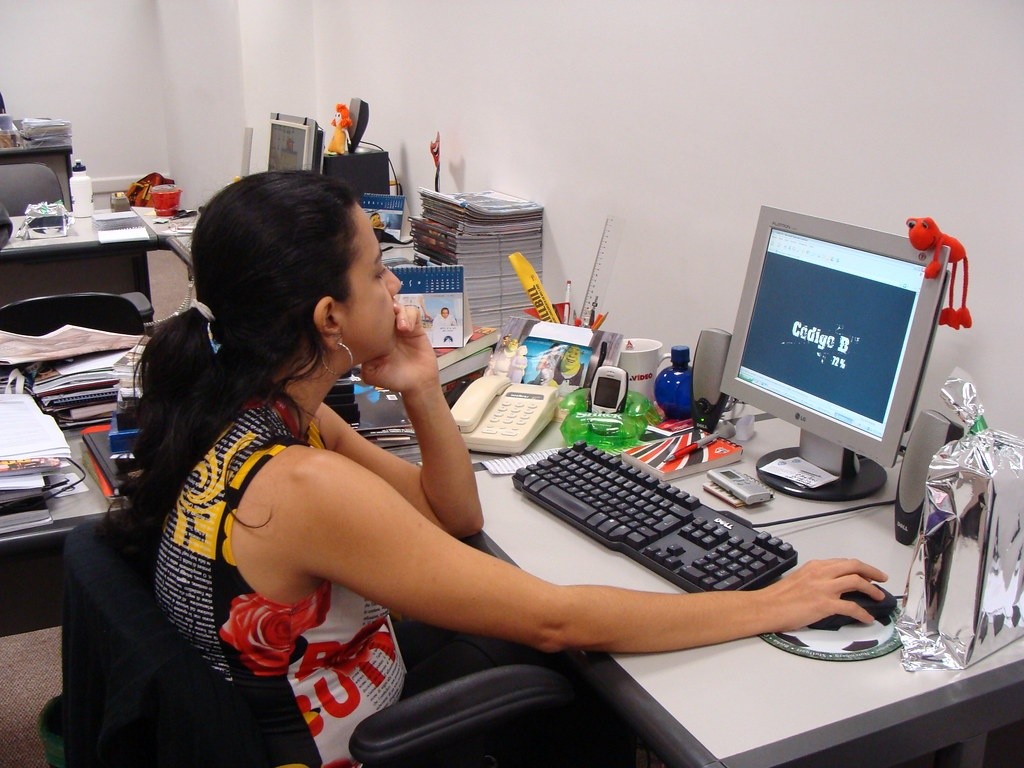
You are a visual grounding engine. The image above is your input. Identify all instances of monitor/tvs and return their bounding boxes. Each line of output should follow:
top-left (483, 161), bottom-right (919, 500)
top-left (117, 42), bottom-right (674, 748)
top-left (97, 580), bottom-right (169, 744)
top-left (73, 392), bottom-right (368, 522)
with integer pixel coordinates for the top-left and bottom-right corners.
top-left (267, 113), bottom-right (323, 179)
top-left (719, 206), bottom-right (952, 502)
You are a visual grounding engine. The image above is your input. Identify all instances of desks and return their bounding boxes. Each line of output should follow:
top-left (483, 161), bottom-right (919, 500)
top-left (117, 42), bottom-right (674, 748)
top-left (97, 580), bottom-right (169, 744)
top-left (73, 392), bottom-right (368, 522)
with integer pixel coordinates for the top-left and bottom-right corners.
top-left (0, 204), bottom-right (1024, 768)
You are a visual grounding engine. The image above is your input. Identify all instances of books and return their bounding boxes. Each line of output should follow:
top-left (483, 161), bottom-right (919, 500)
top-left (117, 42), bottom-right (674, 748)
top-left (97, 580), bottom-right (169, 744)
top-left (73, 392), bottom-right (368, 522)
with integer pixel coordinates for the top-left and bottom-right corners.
top-left (0, 310), bottom-right (500, 536)
top-left (621, 428), bottom-right (743, 482)
top-left (408, 187), bottom-right (546, 346)
top-left (29, 215), bottom-right (66, 238)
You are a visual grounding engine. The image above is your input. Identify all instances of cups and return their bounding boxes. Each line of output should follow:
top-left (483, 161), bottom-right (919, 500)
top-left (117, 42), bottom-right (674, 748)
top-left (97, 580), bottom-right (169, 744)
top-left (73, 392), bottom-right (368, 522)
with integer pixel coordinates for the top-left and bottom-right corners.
top-left (149, 188), bottom-right (183, 216)
top-left (617, 338), bottom-right (671, 405)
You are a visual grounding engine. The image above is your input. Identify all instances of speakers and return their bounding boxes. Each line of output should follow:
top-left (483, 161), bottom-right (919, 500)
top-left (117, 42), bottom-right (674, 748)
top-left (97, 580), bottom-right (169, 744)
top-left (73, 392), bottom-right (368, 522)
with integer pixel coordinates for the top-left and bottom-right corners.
top-left (895, 409), bottom-right (964, 546)
top-left (690, 329), bottom-right (732, 435)
top-left (344, 97), bottom-right (369, 153)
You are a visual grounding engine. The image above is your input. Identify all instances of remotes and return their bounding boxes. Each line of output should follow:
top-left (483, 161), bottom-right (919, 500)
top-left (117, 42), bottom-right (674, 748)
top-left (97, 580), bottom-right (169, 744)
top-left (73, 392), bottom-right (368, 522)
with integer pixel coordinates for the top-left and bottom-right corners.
top-left (707, 468), bottom-right (770, 505)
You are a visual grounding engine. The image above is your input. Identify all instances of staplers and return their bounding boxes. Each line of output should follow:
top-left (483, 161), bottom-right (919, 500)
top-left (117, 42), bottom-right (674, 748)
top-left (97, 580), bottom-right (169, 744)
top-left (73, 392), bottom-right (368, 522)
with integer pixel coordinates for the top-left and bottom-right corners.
top-left (172, 209), bottom-right (197, 220)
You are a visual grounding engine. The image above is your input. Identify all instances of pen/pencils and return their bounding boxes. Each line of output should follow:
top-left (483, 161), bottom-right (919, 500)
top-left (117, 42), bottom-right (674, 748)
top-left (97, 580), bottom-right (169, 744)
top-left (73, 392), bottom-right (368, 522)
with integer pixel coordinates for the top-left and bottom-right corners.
top-left (564, 281), bottom-right (571, 325)
top-left (663, 431), bottom-right (721, 463)
top-left (593, 311), bottom-right (609, 331)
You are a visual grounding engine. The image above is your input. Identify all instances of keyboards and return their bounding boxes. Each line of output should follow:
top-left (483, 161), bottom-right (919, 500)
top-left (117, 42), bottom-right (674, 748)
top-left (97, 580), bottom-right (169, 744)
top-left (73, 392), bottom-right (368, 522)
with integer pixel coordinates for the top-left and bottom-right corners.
top-left (511, 440), bottom-right (798, 594)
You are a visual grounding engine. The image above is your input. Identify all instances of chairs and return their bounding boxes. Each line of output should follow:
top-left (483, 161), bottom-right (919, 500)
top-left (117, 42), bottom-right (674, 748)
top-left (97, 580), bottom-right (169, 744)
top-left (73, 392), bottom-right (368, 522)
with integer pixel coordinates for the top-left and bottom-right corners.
top-left (0, 289), bottom-right (154, 337)
top-left (0, 163), bottom-right (65, 216)
top-left (41, 515), bottom-right (637, 768)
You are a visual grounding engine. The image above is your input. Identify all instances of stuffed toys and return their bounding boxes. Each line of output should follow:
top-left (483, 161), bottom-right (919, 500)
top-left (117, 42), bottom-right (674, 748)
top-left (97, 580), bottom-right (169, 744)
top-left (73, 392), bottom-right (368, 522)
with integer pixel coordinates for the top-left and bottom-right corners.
top-left (906, 217), bottom-right (972, 331)
top-left (326, 103), bottom-right (352, 155)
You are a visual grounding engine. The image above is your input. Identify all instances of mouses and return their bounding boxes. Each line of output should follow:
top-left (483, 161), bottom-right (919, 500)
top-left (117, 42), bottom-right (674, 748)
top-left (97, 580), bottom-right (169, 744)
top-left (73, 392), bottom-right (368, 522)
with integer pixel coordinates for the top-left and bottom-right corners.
top-left (806, 584), bottom-right (898, 630)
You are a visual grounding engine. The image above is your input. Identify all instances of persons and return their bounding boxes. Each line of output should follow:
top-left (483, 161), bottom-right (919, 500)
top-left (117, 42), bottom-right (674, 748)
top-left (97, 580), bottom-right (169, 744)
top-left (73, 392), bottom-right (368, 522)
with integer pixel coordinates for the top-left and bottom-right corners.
top-left (432, 308), bottom-right (458, 327)
top-left (370, 213), bottom-right (384, 228)
top-left (394, 294), bottom-right (431, 322)
top-left (117, 169), bottom-right (887, 768)
top-left (536, 343), bottom-right (563, 385)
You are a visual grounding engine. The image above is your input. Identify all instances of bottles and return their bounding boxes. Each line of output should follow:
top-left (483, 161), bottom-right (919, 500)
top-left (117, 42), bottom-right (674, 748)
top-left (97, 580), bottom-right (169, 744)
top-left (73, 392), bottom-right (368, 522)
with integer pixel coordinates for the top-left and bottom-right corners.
top-left (654, 346), bottom-right (693, 419)
top-left (69, 160), bottom-right (93, 218)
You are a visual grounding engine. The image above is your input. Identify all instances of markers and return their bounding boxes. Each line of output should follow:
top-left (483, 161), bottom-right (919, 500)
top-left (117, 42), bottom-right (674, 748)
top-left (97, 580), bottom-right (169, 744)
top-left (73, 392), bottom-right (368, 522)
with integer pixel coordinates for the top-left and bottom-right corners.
top-left (508, 252), bottom-right (561, 324)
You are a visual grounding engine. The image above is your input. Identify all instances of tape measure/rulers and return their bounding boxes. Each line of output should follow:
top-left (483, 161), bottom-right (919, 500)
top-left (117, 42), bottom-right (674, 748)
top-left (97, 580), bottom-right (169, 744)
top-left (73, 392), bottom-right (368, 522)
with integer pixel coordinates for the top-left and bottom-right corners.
top-left (578, 216), bottom-right (625, 327)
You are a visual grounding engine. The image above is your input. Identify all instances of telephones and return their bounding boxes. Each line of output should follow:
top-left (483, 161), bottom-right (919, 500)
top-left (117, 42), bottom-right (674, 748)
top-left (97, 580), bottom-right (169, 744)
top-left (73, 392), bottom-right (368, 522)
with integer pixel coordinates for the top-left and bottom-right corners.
top-left (450, 375), bottom-right (560, 454)
